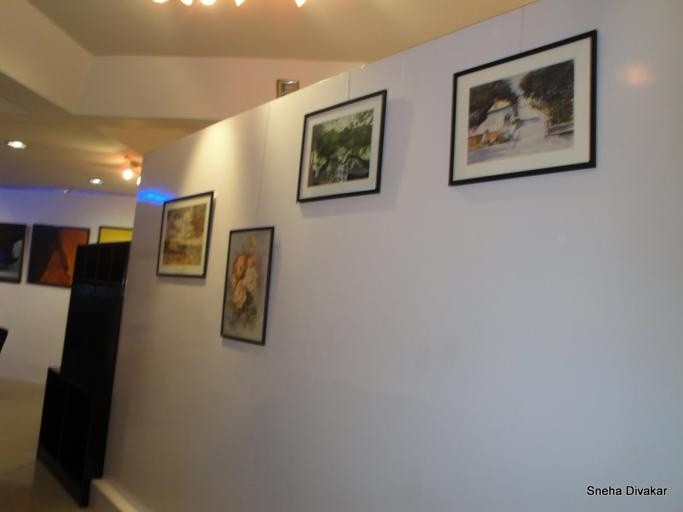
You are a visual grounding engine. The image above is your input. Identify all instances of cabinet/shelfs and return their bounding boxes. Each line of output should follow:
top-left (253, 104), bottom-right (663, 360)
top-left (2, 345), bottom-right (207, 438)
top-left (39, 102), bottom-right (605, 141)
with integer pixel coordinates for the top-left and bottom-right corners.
top-left (37, 242), bottom-right (131, 501)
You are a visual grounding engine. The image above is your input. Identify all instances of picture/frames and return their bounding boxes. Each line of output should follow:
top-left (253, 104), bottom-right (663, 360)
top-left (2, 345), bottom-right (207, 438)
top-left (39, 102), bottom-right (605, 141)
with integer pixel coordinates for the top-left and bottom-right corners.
top-left (156, 191), bottom-right (217, 277)
top-left (447, 29), bottom-right (597, 186)
top-left (28, 224), bottom-right (90, 288)
top-left (88, 226), bottom-right (133, 288)
top-left (220, 228), bottom-right (276, 345)
top-left (296, 90), bottom-right (388, 203)
top-left (0, 223), bottom-right (25, 284)
